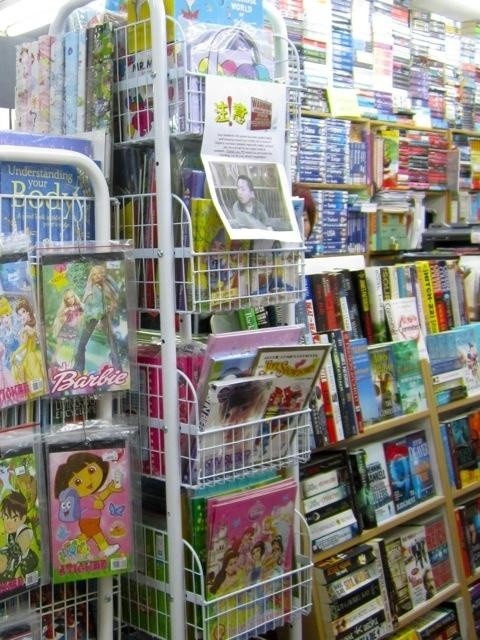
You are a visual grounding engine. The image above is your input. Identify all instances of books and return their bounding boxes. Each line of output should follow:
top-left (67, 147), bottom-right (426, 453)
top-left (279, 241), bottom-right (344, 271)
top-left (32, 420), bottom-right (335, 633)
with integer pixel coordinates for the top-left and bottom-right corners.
top-left (0, 0), bottom-right (480, 639)
